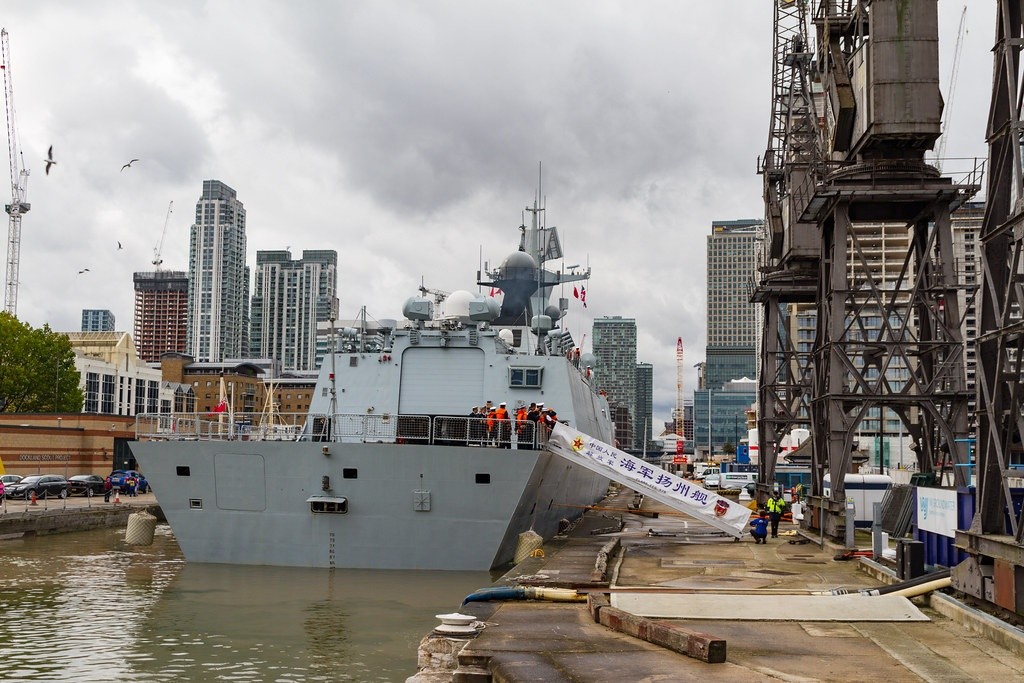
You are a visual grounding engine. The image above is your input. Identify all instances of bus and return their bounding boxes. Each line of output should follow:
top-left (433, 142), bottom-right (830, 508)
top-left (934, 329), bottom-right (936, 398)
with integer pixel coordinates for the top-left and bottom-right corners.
top-left (718, 472), bottom-right (759, 492)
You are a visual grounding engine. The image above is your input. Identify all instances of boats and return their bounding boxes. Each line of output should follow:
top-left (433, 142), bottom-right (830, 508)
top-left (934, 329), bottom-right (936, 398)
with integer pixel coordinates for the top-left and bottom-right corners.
top-left (130, 154), bottom-right (621, 570)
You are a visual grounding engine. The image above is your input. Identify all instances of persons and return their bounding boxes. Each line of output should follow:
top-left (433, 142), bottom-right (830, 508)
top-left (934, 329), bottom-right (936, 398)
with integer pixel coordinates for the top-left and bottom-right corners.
top-left (749, 510), bottom-right (768, 544)
top-left (104, 477), bottom-right (112, 501)
top-left (468, 400), bottom-right (557, 450)
top-left (125, 476), bottom-right (147, 497)
top-left (567, 346), bottom-right (606, 396)
top-left (0, 480), bottom-right (5, 507)
top-left (766, 491), bottom-right (785, 538)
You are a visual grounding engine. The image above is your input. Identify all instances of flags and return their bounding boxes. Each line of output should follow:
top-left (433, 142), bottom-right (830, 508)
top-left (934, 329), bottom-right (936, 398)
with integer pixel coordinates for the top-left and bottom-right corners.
top-left (490, 286), bottom-right (494, 297)
top-left (574, 287), bottom-right (578, 298)
top-left (208, 396), bottom-right (226, 416)
top-left (495, 288), bottom-right (501, 295)
top-left (581, 285), bottom-right (587, 307)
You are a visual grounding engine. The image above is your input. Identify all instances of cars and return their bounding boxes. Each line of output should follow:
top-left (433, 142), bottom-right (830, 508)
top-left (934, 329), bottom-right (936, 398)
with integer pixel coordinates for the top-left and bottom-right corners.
top-left (0, 475), bottom-right (25, 491)
top-left (703, 475), bottom-right (720, 489)
top-left (695, 467), bottom-right (720, 479)
top-left (107, 469), bottom-right (150, 495)
top-left (67, 475), bottom-right (112, 497)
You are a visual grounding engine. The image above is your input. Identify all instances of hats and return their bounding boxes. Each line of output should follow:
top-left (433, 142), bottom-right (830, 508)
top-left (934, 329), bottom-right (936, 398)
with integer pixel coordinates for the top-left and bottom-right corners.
top-left (541, 407), bottom-right (549, 411)
top-left (490, 406), bottom-right (497, 411)
top-left (773, 491), bottom-right (778, 495)
top-left (473, 406), bottom-right (478, 409)
top-left (536, 403), bottom-right (544, 408)
top-left (500, 402), bottom-right (506, 407)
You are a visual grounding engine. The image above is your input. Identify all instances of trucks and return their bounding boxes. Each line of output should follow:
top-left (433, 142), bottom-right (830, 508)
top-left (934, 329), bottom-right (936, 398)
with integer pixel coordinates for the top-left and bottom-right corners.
top-left (693, 462), bottom-right (716, 475)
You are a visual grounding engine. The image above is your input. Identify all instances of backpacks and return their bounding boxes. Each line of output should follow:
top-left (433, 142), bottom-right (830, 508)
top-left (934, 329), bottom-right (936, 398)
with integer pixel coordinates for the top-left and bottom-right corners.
top-left (105, 482), bottom-right (111, 490)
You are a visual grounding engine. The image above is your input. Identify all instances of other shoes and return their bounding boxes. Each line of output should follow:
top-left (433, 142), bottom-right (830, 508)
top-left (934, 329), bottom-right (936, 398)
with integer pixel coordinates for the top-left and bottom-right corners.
top-left (772, 534), bottom-right (778, 538)
top-left (763, 540), bottom-right (766, 544)
top-left (756, 539), bottom-right (761, 544)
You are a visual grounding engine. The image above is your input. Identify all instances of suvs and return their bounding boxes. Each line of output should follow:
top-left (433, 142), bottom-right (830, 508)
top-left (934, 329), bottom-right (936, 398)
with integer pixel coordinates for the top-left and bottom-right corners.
top-left (5, 475), bottom-right (68, 500)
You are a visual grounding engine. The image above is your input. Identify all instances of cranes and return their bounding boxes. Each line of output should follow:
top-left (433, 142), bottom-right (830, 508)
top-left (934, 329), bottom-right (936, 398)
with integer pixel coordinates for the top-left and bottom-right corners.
top-left (676, 336), bottom-right (685, 436)
top-left (0, 25), bottom-right (31, 321)
top-left (150, 200), bottom-right (176, 273)
top-left (748, 0), bottom-right (1024, 584)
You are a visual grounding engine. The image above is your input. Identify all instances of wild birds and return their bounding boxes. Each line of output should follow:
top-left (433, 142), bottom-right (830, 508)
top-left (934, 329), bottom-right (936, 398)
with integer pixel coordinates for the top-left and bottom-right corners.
top-left (79, 269), bottom-right (89, 273)
top-left (117, 241), bottom-right (122, 249)
top-left (45, 145), bottom-right (56, 175)
top-left (120, 159), bottom-right (140, 170)
